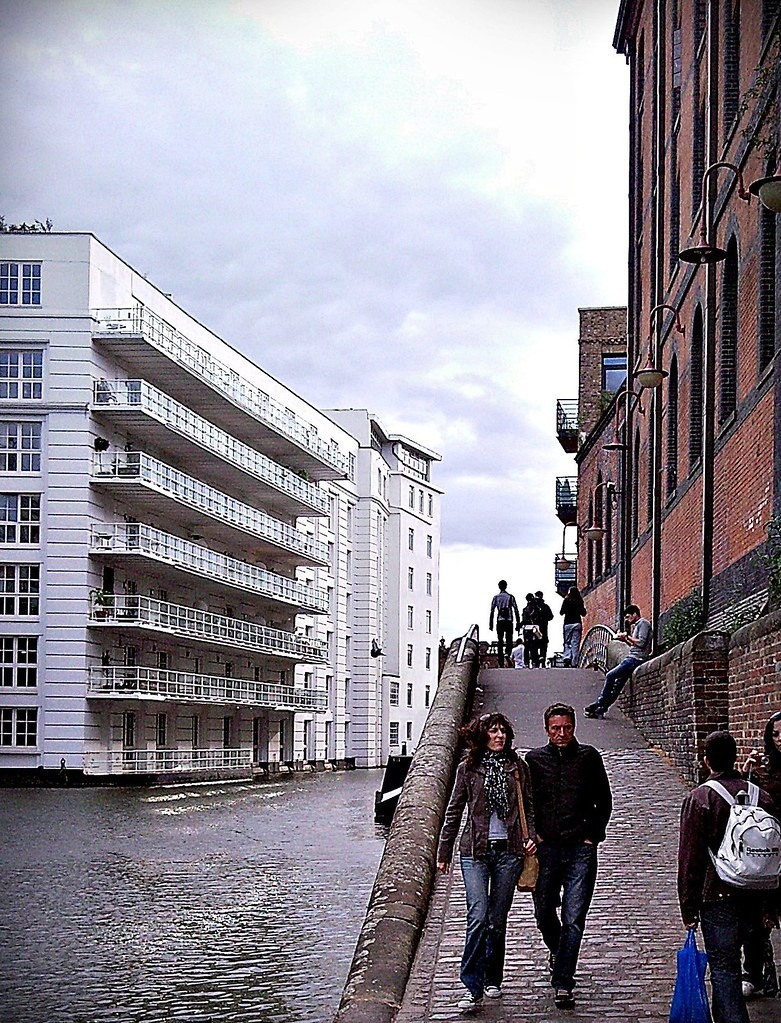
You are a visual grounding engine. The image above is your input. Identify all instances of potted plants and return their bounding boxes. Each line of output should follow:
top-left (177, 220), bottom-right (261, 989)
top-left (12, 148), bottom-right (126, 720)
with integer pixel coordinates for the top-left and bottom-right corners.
top-left (103, 650), bottom-right (111, 694)
top-left (89, 586), bottom-right (114, 622)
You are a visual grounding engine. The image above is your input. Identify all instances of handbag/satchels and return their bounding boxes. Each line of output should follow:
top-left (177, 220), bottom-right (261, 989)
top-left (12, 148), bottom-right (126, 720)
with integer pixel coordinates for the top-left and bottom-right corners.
top-left (524, 624), bottom-right (539, 638)
top-left (500, 607), bottom-right (509, 618)
top-left (669, 930), bottom-right (713, 1023)
top-left (517, 854), bottom-right (539, 892)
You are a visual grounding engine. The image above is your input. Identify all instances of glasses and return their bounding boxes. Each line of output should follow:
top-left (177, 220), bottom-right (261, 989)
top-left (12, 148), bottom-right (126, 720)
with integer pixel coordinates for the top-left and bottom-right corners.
top-left (476, 712), bottom-right (501, 735)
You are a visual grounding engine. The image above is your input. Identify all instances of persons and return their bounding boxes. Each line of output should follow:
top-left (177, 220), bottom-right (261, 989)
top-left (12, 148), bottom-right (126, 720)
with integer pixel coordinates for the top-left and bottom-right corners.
top-left (524, 702), bottom-right (612, 1007)
top-left (560, 586), bottom-right (587, 667)
top-left (511, 591), bottom-right (554, 668)
top-left (490, 580), bottom-right (520, 668)
top-left (437, 712), bottom-right (537, 1008)
top-left (583, 604), bottom-right (653, 719)
top-left (740, 711), bottom-right (781, 1000)
top-left (678, 731), bottom-right (781, 1023)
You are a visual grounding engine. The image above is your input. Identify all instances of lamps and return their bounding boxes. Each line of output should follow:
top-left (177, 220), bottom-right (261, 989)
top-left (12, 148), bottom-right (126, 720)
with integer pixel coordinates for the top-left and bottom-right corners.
top-left (752, 162), bottom-right (781, 211)
top-left (678, 161), bottom-right (751, 264)
top-left (583, 483), bottom-right (618, 540)
top-left (554, 521), bottom-right (587, 570)
top-left (633, 304), bottom-right (685, 388)
top-left (603, 389), bottom-right (645, 451)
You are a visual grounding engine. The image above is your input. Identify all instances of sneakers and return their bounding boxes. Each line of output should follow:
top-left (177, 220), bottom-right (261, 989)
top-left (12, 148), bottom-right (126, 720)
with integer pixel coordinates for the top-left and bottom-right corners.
top-left (484, 986), bottom-right (502, 998)
top-left (458, 990), bottom-right (482, 1008)
top-left (547, 951), bottom-right (555, 973)
top-left (555, 989), bottom-right (575, 1005)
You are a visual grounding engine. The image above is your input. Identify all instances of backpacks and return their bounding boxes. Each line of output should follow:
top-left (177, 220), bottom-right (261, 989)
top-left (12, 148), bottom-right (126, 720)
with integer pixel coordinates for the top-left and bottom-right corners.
top-left (699, 779), bottom-right (781, 890)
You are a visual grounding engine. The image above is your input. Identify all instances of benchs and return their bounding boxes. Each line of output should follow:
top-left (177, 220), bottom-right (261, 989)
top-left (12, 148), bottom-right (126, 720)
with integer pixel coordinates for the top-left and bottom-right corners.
top-left (251, 756), bottom-right (356, 780)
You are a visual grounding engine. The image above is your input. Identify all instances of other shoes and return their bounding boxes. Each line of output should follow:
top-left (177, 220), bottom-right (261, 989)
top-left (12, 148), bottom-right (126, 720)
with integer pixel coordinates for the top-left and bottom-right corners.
top-left (532, 663), bottom-right (538, 668)
top-left (523, 664), bottom-right (528, 668)
top-left (500, 665), bottom-right (505, 668)
top-left (571, 664), bottom-right (579, 668)
top-left (504, 654), bottom-right (513, 667)
top-left (763, 989), bottom-right (781, 999)
top-left (583, 712), bottom-right (603, 720)
top-left (563, 659), bottom-right (571, 668)
top-left (744, 988), bottom-right (764, 1002)
top-left (584, 702), bottom-right (597, 712)
